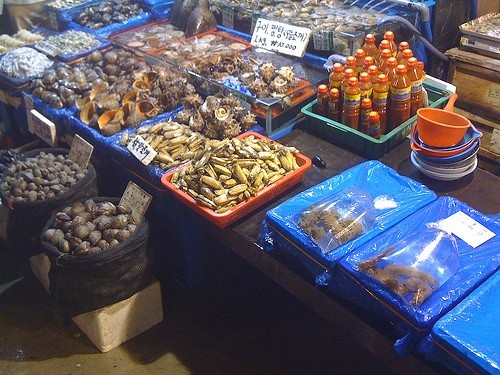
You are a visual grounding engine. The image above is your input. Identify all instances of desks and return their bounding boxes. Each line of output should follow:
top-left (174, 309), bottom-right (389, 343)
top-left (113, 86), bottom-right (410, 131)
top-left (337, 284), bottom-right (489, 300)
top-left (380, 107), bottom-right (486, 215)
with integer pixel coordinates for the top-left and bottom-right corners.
top-left (174, 128), bottom-right (500, 375)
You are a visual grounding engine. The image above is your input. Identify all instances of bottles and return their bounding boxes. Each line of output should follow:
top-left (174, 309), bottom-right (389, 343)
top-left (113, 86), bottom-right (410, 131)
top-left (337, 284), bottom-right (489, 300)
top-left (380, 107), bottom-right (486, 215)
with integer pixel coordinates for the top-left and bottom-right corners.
top-left (315, 31), bottom-right (425, 140)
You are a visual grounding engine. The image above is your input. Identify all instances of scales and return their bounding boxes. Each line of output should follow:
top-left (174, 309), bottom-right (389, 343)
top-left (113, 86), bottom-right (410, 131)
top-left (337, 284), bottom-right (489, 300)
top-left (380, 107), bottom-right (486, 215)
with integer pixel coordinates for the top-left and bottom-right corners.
top-left (458, 12), bottom-right (500, 59)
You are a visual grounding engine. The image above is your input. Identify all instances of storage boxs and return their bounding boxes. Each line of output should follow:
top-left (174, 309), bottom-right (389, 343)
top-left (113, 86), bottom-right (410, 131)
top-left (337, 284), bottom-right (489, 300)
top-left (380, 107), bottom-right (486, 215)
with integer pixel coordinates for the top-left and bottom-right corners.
top-left (0, 0), bottom-right (452, 188)
top-left (29, 246), bottom-right (164, 354)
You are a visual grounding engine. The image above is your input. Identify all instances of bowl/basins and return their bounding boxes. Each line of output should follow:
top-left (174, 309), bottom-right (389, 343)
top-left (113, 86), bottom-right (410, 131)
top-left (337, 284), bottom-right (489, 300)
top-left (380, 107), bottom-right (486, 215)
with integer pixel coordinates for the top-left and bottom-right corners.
top-left (408, 107), bottom-right (483, 181)
